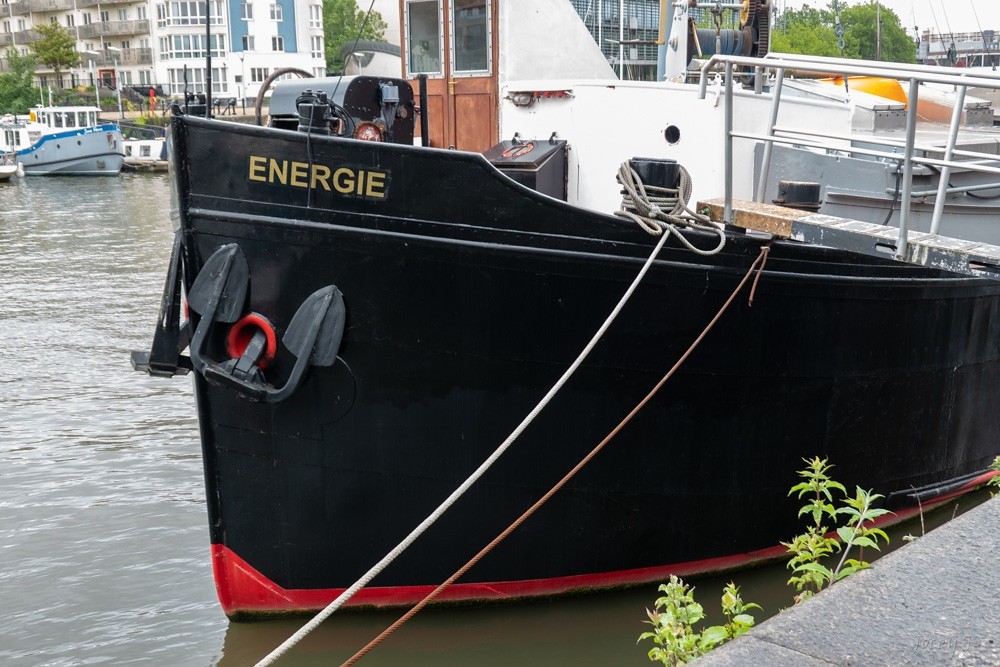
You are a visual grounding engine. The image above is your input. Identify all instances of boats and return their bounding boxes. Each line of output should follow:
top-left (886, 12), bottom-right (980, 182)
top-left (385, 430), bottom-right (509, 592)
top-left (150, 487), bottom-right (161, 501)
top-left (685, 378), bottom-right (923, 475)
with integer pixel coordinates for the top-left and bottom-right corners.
top-left (0, 153), bottom-right (17, 179)
top-left (0, 77), bottom-right (127, 175)
top-left (121, 135), bottom-right (168, 172)
top-left (130, 0), bottom-right (1000, 620)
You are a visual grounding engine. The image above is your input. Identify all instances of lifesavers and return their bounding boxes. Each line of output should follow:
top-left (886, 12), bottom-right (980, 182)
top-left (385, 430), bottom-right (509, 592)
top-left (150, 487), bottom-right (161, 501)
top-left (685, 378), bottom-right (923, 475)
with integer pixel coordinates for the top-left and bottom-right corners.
top-left (150, 97), bottom-right (156, 104)
top-left (29, 112), bottom-right (36, 122)
